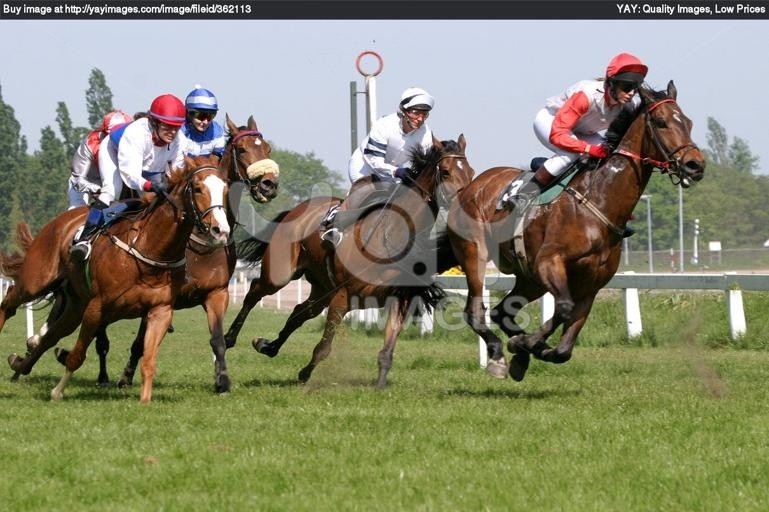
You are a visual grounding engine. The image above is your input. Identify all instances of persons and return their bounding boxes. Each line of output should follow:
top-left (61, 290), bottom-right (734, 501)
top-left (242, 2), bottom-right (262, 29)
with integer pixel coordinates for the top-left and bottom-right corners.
top-left (70, 93), bottom-right (185, 263)
top-left (326, 88), bottom-right (434, 243)
top-left (70, 110), bottom-right (135, 211)
top-left (507, 52), bottom-right (648, 208)
top-left (169, 88), bottom-right (225, 174)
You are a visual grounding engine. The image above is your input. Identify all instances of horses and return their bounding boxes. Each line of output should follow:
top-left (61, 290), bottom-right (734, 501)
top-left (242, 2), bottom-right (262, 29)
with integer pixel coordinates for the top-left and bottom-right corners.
top-left (96, 111), bottom-right (279, 397)
top-left (0, 192), bottom-right (175, 357)
top-left (382, 78), bottom-right (707, 384)
top-left (6, 149), bottom-right (231, 405)
top-left (222, 129), bottom-right (476, 389)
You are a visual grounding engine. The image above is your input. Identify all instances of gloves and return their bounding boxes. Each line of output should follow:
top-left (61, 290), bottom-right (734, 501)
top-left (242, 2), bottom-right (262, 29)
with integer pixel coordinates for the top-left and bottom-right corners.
top-left (143, 181), bottom-right (161, 194)
top-left (394, 168), bottom-right (413, 182)
top-left (585, 145), bottom-right (607, 159)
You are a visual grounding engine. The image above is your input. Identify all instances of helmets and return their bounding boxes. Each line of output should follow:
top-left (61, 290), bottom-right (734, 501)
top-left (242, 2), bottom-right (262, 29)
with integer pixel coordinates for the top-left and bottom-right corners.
top-left (186, 89), bottom-right (218, 111)
top-left (150, 95), bottom-right (185, 126)
top-left (400, 87), bottom-right (434, 111)
top-left (103, 110), bottom-right (131, 134)
top-left (607, 53), bottom-right (648, 83)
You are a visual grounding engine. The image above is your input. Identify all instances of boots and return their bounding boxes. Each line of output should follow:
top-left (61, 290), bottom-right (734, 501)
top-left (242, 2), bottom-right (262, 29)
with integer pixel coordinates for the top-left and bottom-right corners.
top-left (320, 206), bottom-right (357, 248)
top-left (507, 164), bottom-right (558, 216)
top-left (69, 199), bottom-right (110, 264)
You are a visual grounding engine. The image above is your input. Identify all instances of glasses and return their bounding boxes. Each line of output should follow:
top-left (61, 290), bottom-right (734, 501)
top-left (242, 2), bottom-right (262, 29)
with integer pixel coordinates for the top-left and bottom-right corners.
top-left (189, 111), bottom-right (215, 121)
top-left (406, 109), bottom-right (429, 119)
top-left (616, 80), bottom-right (637, 94)
top-left (158, 124), bottom-right (180, 132)
